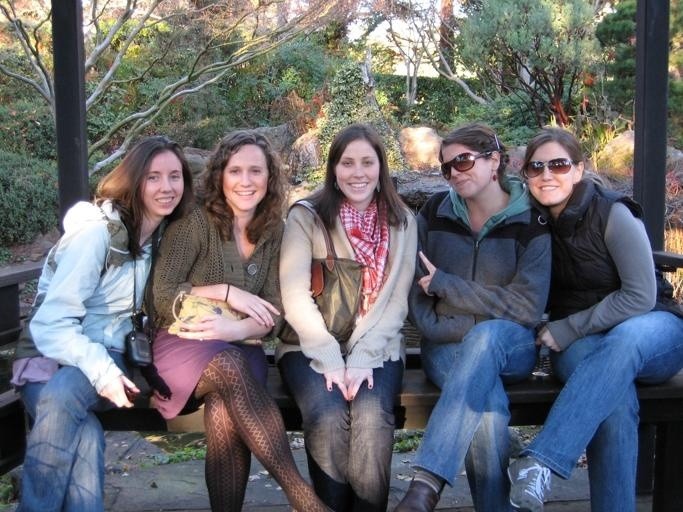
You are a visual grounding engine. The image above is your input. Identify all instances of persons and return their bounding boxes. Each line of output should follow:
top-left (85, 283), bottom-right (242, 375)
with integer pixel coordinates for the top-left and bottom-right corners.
top-left (503, 126), bottom-right (681, 512)
top-left (6, 135), bottom-right (196, 512)
top-left (278, 122), bottom-right (418, 512)
top-left (141, 131), bottom-right (337, 512)
top-left (388, 122), bottom-right (556, 512)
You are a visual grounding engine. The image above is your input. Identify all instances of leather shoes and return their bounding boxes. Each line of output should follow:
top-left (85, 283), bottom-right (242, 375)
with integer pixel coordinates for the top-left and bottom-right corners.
top-left (391, 479), bottom-right (441, 511)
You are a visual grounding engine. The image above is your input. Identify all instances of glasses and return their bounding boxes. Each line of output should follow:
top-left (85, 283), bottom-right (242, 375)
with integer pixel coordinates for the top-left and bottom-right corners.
top-left (442, 151), bottom-right (493, 182)
top-left (524, 158), bottom-right (575, 178)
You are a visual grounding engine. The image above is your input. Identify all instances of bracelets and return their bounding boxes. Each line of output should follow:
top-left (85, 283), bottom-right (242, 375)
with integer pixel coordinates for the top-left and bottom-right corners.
top-left (223, 283), bottom-right (231, 303)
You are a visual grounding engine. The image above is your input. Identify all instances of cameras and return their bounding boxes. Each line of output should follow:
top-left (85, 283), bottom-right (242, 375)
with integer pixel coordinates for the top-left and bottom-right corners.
top-left (125, 313), bottom-right (153, 368)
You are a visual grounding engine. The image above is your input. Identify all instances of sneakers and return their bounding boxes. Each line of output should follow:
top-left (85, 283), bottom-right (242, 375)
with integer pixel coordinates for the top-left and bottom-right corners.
top-left (506, 457), bottom-right (552, 511)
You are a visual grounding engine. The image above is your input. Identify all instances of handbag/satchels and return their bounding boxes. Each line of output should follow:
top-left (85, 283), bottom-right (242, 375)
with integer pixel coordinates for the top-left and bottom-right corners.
top-left (277, 257), bottom-right (364, 346)
top-left (168, 291), bottom-right (263, 346)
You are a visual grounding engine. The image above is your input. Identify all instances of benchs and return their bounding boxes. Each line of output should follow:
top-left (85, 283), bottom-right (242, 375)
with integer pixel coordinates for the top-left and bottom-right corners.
top-left (24, 352), bottom-right (681, 510)
